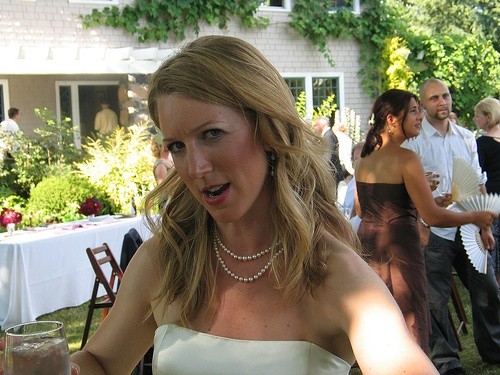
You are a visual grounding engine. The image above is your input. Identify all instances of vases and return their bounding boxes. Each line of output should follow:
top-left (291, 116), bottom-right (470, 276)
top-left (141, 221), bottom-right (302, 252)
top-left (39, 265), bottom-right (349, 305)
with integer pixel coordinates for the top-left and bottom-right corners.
top-left (88, 214), bottom-right (94, 221)
top-left (6, 223), bottom-right (15, 235)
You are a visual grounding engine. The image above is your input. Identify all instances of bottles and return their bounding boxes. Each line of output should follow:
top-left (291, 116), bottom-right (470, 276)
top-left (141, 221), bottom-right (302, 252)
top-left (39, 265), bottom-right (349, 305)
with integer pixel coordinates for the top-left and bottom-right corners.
top-left (130, 198), bottom-right (136, 218)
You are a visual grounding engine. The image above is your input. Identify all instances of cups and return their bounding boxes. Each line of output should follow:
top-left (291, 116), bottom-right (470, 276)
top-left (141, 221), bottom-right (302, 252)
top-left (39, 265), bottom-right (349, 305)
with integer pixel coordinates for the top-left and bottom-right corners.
top-left (8, 224), bottom-right (15, 235)
top-left (5, 321), bottom-right (69, 375)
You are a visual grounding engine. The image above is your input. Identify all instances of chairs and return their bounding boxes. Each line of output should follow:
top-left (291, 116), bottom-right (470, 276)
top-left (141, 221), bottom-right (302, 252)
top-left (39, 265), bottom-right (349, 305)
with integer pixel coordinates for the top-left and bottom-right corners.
top-left (80, 242), bottom-right (124, 350)
top-left (116, 228), bottom-right (143, 293)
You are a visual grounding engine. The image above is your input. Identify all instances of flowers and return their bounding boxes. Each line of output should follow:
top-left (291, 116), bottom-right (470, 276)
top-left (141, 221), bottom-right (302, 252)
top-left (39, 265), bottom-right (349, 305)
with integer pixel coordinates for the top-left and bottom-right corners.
top-left (75, 196), bottom-right (102, 216)
top-left (0, 207), bottom-right (22, 228)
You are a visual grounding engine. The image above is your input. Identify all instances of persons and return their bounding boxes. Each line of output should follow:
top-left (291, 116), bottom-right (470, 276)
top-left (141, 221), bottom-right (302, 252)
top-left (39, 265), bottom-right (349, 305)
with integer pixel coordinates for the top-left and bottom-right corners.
top-left (95, 98), bottom-right (118, 136)
top-left (0, 36), bottom-right (441, 375)
top-left (0, 107), bottom-right (22, 160)
top-left (304, 78), bottom-right (500, 375)
top-left (150, 136), bottom-right (175, 210)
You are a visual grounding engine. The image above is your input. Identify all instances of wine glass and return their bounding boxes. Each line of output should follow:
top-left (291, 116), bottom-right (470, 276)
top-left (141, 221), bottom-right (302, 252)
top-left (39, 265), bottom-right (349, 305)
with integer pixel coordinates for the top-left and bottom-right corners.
top-left (436, 177), bottom-right (451, 206)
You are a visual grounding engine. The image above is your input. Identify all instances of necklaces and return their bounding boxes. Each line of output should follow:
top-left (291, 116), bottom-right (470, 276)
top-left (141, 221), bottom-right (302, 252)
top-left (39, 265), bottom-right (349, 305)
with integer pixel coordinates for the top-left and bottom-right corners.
top-left (212, 223), bottom-right (284, 283)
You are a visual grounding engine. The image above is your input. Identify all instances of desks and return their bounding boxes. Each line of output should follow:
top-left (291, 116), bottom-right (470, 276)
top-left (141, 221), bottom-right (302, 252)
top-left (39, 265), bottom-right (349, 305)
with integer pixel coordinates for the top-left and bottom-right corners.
top-left (0, 214), bottom-right (162, 332)
